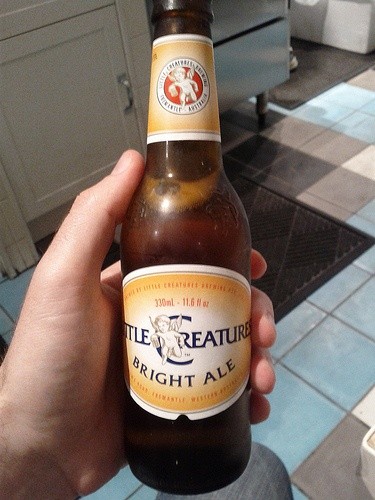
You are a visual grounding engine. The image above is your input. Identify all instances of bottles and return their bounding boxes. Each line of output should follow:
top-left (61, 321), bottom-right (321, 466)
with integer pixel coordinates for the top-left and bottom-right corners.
top-left (117, 0), bottom-right (252, 496)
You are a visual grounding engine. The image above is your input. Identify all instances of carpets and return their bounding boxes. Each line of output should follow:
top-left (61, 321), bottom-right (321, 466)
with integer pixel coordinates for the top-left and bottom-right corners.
top-left (268, 36), bottom-right (375, 110)
top-left (35, 169), bottom-right (375, 326)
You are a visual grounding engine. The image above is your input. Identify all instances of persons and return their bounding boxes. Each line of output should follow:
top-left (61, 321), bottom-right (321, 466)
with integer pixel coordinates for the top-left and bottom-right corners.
top-left (0, 150), bottom-right (295, 500)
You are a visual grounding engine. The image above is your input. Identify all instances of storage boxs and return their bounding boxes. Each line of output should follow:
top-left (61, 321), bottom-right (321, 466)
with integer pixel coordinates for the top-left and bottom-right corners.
top-left (290, 0), bottom-right (374, 56)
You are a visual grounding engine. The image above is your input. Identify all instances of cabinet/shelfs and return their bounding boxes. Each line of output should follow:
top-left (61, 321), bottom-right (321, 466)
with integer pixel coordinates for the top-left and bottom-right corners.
top-left (0, 0), bottom-right (290, 283)
